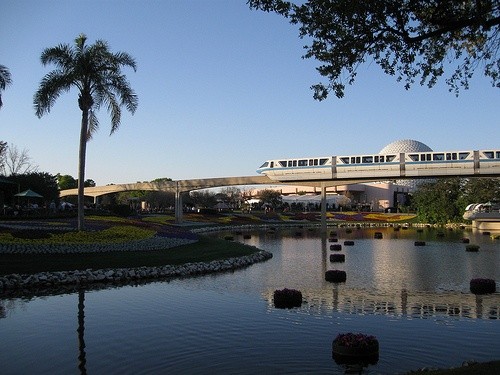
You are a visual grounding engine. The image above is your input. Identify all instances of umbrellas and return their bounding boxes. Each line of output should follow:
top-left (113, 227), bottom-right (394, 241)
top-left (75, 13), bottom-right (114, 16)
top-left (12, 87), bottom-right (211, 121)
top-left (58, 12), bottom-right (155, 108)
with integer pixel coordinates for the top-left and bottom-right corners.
top-left (14, 189), bottom-right (43, 208)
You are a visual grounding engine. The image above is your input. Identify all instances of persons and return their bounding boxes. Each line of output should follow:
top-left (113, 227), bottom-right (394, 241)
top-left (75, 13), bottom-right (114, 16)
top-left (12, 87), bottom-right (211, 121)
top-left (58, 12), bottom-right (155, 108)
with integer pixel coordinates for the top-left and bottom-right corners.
top-left (3, 201), bottom-right (38, 218)
top-left (50, 200), bottom-right (58, 222)
